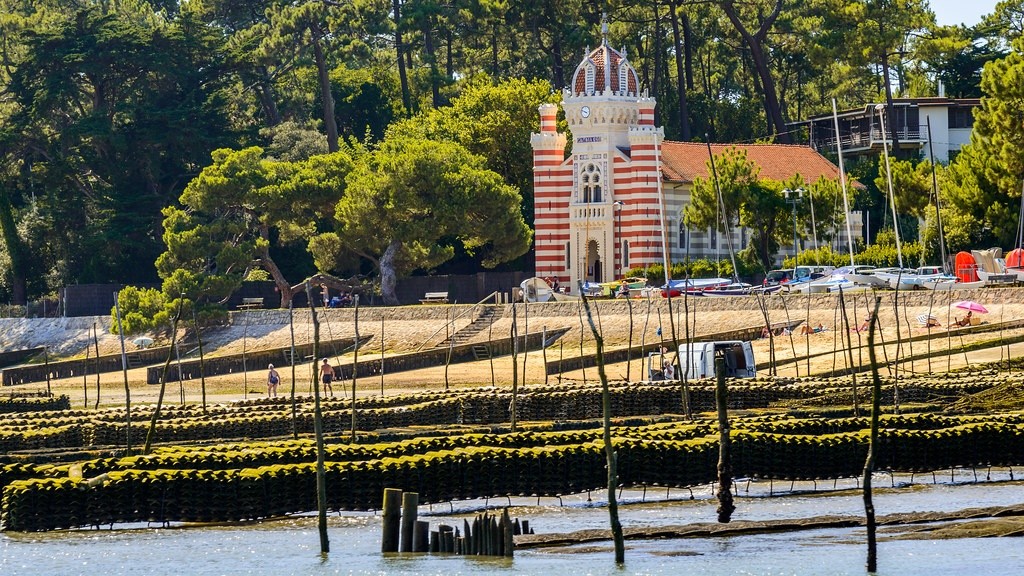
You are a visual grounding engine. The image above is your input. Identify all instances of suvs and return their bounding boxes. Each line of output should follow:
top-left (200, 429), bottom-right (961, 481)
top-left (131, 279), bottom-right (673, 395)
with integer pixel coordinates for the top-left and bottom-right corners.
top-left (763, 268), bottom-right (794, 290)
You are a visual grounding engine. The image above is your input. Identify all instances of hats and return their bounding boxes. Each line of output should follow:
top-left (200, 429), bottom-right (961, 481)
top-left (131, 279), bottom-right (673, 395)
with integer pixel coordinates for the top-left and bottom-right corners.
top-left (553, 276), bottom-right (557, 278)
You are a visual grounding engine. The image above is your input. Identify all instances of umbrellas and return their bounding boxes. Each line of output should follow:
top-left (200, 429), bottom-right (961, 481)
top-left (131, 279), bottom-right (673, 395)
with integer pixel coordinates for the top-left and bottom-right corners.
top-left (916, 315), bottom-right (941, 326)
top-left (951, 302), bottom-right (988, 315)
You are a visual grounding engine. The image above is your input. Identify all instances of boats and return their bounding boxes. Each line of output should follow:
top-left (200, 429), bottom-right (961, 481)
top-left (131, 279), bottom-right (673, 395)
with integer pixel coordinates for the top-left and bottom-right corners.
top-left (519, 277), bottom-right (580, 301)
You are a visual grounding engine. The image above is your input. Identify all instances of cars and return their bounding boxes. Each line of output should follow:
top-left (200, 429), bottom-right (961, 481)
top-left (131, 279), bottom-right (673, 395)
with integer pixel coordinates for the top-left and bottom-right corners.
top-left (817, 265), bottom-right (876, 276)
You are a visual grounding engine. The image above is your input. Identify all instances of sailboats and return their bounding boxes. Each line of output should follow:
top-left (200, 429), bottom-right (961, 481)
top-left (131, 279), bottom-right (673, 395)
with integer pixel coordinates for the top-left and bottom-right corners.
top-left (550, 98), bottom-right (1023, 298)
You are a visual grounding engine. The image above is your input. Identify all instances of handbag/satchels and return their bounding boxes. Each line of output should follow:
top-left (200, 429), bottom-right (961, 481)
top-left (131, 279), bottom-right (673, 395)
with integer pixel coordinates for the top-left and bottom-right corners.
top-left (270, 377), bottom-right (278, 385)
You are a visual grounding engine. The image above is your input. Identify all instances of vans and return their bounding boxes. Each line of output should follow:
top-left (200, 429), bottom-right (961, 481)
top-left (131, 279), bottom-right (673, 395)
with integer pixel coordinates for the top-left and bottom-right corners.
top-left (793, 264), bottom-right (836, 278)
top-left (647, 340), bottom-right (757, 381)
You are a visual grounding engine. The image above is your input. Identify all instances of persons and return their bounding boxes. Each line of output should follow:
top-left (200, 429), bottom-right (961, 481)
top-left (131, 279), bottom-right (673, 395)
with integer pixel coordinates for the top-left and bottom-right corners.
top-left (319, 358), bottom-right (335, 396)
top-left (858, 309), bottom-right (873, 332)
top-left (665, 360), bottom-right (674, 380)
top-left (554, 276), bottom-right (560, 293)
top-left (801, 324), bottom-right (822, 334)
top-left (319, 283), bottom-right (329, 307)
top-left (267, 364), bottom-right (281, 396)
top-left (328, 292), bottom-right (352, 307)
top-left (949, 312), bottom-right (972, 326)
top-left (760, 325), bottom-right (775, 339)
top-left (545, 277), bottom-right (553, 289)
top-left (616, 281), bottom-right (629, 299)
